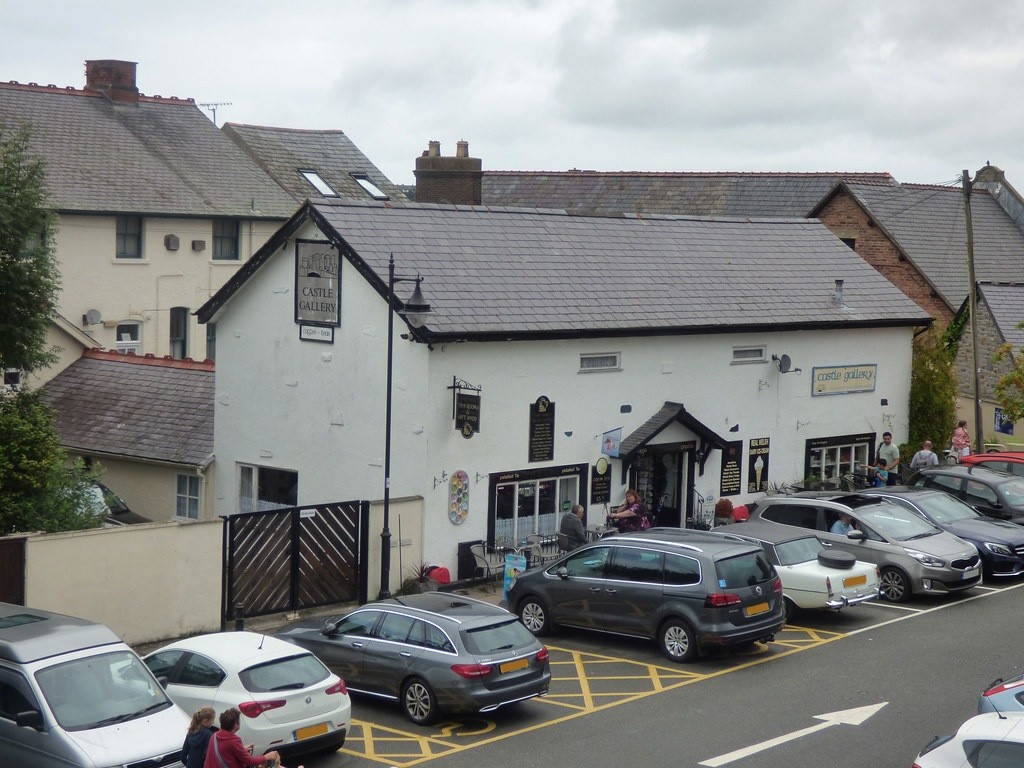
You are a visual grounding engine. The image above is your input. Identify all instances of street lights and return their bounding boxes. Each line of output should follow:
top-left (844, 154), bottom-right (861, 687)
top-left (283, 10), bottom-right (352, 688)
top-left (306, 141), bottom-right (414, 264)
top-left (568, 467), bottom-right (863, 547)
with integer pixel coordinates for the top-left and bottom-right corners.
top-left (374, 250), bottom-right (436, 600)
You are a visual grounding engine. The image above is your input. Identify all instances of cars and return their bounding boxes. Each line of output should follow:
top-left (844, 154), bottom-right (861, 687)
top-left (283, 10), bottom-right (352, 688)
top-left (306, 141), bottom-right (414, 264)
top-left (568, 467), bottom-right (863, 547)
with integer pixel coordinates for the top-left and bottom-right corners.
top-left (107, 631), bottom-right (351, 763)
top-left (52, 475), bottom-right (153, 529)
top-left (956, 451), bottom-right (1024, 479)
top-left (977, 672), bottom-right (1024, 712)
top-left (901, 462), bottom-right (1024, 526)
top-left (910, 711), bottom-right (1024, 768)
top-left (709, 521), bottom-right (882, 625)
top-left (853, 485), bottom-right (1024, 582)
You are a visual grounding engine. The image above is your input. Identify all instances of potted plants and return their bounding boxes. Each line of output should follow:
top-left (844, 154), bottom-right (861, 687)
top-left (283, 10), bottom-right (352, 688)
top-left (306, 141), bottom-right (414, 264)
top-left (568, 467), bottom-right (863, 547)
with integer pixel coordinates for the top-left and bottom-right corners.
top-left (401, 559), bottom-right (440, 593)
top-left (714, 497), bottom-right (735, 526)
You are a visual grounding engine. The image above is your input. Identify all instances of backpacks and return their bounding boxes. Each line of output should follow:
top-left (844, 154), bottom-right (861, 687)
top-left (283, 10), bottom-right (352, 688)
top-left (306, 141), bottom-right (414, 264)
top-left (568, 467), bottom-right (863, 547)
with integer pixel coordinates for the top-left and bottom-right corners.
top-left (948, 445), bottom-right (959, 464)
top-left (915, 452), bottom-right (933, 472)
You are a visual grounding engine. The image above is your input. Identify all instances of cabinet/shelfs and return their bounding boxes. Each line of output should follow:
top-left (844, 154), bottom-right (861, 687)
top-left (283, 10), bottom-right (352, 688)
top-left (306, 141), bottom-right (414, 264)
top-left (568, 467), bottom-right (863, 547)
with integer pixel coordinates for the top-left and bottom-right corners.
top-left (637, 469), bottom-right (654, 509)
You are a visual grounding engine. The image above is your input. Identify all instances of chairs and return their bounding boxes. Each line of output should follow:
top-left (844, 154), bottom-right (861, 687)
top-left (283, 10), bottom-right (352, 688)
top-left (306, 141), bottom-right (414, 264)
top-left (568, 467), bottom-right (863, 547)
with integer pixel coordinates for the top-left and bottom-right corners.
top-left (781, 481), bottom-right (799, 495)
top-left (648, 514), bottom-right (656, 528)
top-left (552, 530), bottom-right (587, 556)
top-left (527, 532), bottom-right (561, 563)
top-left (843, 473), bottom-right (866, 489)
top-left (765, 488), bottom-right (780, 496)
top-left (469, 543), bottom-right (508, 593)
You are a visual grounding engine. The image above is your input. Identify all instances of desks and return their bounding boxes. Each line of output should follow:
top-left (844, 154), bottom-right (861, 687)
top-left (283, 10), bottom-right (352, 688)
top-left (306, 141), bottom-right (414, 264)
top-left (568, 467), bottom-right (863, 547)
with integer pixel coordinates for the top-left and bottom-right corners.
top-left (584, 525), bottom-right (619, 542)
top-left (495, 539), bottom-right (542, 584)
top-left (850, 471), bottom-right (877, 488)
top-left (791, 479), bottom-right (845, 492)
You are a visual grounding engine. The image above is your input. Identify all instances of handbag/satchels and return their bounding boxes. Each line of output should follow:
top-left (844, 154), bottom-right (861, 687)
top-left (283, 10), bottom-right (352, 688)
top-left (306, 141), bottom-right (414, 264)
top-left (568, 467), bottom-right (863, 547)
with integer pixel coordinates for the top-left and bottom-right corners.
top-left (871, 471), bottom-right (881, 487)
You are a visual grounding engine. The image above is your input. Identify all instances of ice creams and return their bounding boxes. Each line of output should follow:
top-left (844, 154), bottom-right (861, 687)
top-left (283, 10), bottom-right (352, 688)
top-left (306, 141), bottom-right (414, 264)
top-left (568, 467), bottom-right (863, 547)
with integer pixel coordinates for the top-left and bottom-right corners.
top-left (755, 455), bottom-right (763, 490)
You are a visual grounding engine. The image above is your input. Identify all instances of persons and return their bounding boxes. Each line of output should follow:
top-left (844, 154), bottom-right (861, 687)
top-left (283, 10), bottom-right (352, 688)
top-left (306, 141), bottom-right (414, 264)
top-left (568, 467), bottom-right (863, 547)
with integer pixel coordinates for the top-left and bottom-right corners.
top-left (860, 458), bottom-right (888, 487)
top-left (878, 431), bottom-right (900, 486)
top-left (910, 440), bottom-right (939, 486)
top-left (204, 708), bottom-right (276, 768)
top-left (952, 419), bottom-right (971, 461)
top-left (181, 707), bottom-right (214, 768)
top-left (829, 512), bottom-right (854, 535)
top-left (607, 488), bottom-right (650, 533)
top-left (558, 505), bottom-right (590, 552)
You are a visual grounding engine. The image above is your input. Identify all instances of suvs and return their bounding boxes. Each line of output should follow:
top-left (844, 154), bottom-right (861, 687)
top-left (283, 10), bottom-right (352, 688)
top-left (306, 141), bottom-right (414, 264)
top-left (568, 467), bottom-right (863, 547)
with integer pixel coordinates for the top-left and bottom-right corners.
top-left (277, 590), bottom-right (552, 726)
top-left (505, 526), bottom-right (787, 663)
top-left (743, 490), bottom-right (983, 602)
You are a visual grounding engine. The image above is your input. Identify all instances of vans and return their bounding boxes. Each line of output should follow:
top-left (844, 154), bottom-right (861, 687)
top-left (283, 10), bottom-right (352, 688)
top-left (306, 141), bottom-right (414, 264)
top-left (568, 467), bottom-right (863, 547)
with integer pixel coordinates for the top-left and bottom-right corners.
top-left (0, 601), bottom-right (195, 768)
